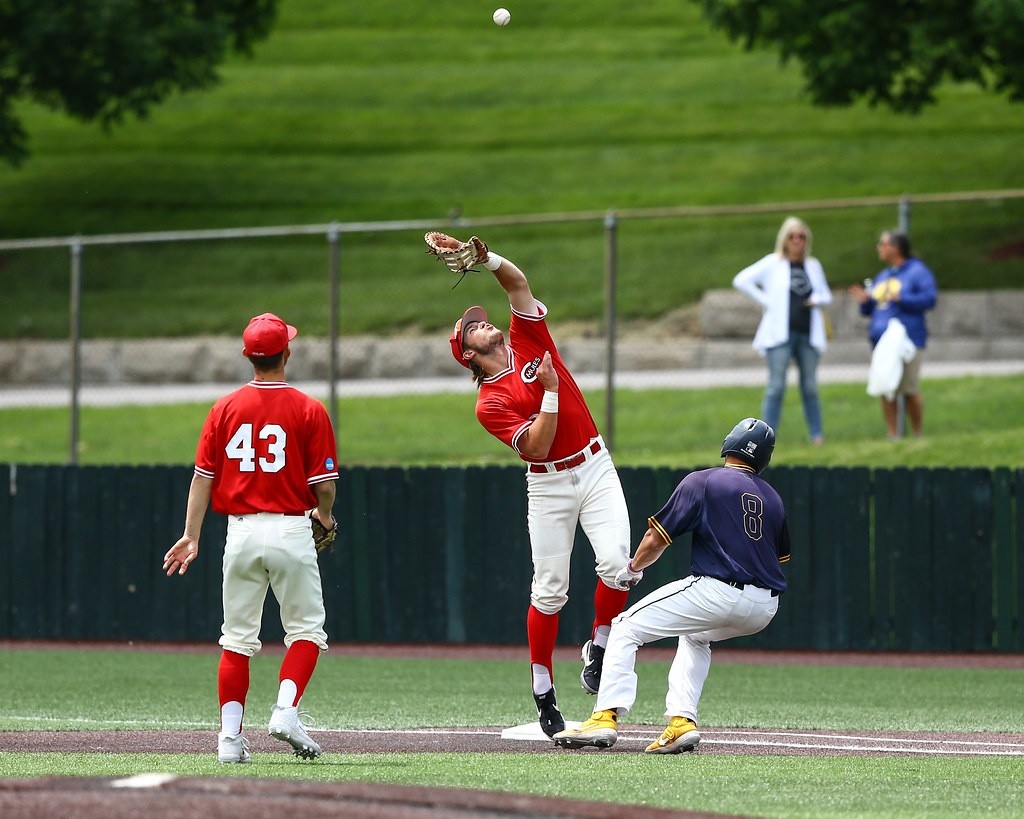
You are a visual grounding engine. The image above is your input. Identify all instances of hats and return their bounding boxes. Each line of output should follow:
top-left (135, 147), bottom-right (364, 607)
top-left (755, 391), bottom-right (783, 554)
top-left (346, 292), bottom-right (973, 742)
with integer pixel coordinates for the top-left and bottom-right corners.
top-left (243, 312), bottom-right (297, 356)
top-left (449, 306), bottom-right (487, 369)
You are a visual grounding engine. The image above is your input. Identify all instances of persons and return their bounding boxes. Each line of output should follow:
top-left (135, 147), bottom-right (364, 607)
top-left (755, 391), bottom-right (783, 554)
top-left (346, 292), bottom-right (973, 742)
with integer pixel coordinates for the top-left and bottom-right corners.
top-left (849, 228), bottom-right (937, 443)
top-left (731, 216), bottom-right (835, 446)
top-left (426, 230), bottom-right (631, 738)
top-left (552, 416), bottom-right (791, 753)
top-left (163, 313), bottom-right (337, 765)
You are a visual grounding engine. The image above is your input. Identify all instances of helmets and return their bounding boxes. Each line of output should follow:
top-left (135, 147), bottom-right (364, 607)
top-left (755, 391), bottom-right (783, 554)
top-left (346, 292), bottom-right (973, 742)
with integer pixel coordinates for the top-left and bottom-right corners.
top-left (720, 417), bottom-right (775, 474)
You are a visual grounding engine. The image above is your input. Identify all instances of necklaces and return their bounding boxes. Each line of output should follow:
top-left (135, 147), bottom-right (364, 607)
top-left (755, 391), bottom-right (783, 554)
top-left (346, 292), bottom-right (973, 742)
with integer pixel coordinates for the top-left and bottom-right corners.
top-left (485, 356), bottom-right (509, 376)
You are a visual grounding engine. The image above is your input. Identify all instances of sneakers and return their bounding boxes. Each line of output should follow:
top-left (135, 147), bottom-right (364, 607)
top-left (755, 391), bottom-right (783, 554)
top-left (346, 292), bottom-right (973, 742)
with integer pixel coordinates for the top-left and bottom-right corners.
top-left (553, 709), bottom-right (618, 749)
top-left (267, 705), bottom-right (322, 760)
top-left (580, 640), bottom-right (605, 695)
top-left (531, 684), bottom-right (565, 740)
top-left (645, 717), bottom-right (701, 754)
top-left (218, 732), bottom-right (252, 764)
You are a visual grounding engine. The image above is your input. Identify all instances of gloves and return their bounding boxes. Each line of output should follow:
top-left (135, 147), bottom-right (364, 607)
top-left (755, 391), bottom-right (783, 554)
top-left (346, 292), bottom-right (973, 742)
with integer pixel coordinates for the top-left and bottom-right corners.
top-left (614, 559), bottom-right (643, 591)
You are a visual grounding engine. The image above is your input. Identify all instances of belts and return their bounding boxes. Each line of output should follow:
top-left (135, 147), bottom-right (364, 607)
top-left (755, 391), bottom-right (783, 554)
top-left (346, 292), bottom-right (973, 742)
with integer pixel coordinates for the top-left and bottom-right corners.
top-left (284, 511), bottom-right (305, 516)
top-left (706, 574), bottom-right (779, 597)
top-left (530, 441), bottom-right (601, 473)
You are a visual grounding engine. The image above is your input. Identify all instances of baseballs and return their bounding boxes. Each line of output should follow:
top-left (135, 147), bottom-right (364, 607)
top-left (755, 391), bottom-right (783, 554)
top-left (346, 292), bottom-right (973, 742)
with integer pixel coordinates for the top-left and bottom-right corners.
top-left (492, 7), bottom-right (511, 27)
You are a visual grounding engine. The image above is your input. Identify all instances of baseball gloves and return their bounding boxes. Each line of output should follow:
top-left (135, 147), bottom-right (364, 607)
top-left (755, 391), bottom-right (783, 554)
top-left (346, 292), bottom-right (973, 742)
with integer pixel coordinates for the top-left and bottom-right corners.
top-left (308, 506), bottom-right (342, 554)
top-left (423, 231), bottom-right (490, 290)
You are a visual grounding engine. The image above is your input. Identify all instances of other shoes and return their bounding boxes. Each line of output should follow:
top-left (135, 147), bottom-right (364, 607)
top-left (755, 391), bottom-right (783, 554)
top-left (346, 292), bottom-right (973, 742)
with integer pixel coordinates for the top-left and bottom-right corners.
top-left (808, 433), bottom-right (825, 444)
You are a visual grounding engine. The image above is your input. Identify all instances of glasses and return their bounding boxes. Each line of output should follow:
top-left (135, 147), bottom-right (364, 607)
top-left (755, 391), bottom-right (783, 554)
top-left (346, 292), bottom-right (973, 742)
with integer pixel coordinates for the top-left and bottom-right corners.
top-left (788, 234), bottom-right (806, 240)
